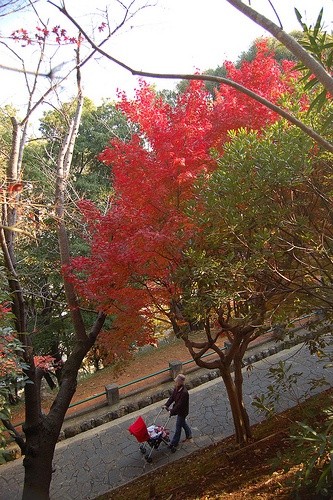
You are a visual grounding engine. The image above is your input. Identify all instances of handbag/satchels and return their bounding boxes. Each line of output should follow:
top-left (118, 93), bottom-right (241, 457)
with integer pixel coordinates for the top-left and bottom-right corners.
top-left (170, 405), bottom-right (177, 415)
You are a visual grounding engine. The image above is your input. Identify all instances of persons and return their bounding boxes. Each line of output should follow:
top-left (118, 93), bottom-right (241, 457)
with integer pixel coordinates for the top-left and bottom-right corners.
top-left (162, 373), bottom-right (193, 452)
top-left (147, 425), bottom-right (165, 441)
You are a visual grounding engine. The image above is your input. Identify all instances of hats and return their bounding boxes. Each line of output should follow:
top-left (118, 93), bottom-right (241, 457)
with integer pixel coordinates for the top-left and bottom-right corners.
top-left (174, 374), bottom-right (186, 385)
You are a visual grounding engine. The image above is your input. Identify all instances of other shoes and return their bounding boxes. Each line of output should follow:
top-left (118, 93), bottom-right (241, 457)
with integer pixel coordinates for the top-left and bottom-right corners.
top-left (168, 441), bottom-right (178, 449)
top-left (183, 436), bottom-right (193, 442)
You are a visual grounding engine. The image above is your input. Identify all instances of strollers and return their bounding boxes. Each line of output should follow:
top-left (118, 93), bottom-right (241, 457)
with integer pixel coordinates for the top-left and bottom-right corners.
top-left (126, 406), bottom-right (183, 463)
